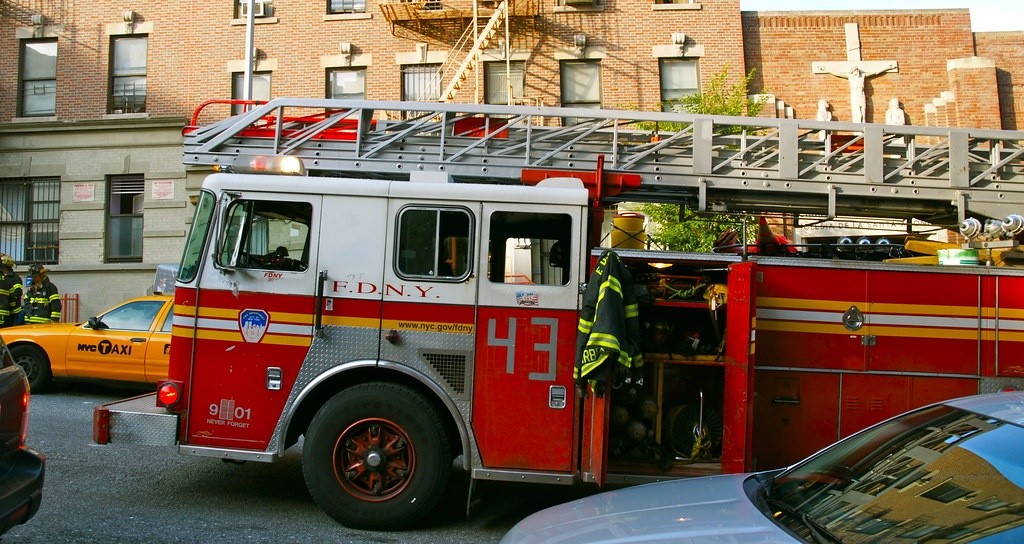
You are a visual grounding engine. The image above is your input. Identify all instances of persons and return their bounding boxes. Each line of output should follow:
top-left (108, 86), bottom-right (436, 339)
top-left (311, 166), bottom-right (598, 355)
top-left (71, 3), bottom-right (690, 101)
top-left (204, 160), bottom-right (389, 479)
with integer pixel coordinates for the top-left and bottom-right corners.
top-left (0, 253), bottom-right (24, 328)
top-left (23, 264), bottom-right (62, 325)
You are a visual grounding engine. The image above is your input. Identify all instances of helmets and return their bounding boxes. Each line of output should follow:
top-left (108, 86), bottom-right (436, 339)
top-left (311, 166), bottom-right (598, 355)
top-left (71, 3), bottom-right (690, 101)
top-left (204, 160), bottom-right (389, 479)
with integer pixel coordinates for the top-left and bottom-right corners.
top-left (548, 240), bottom-right (572, 268)
top-left (28, 264), bottom-right (49, 278)
top-left (0, 253), bottom-right (17, 269)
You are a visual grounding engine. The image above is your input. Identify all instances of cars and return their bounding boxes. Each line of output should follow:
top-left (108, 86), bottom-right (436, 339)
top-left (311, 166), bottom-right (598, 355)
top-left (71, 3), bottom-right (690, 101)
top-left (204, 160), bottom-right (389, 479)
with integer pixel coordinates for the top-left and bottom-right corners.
top-left (495, 390), bottom-right (1023, 544)
top-left (0, 296), bottom-right (175, 393)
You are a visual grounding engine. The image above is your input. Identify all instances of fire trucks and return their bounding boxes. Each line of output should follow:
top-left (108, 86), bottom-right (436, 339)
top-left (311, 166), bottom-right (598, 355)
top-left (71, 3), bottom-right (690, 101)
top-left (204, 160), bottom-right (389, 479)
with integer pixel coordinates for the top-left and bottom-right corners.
top-left (154, 96), bottom-right (1023, 533)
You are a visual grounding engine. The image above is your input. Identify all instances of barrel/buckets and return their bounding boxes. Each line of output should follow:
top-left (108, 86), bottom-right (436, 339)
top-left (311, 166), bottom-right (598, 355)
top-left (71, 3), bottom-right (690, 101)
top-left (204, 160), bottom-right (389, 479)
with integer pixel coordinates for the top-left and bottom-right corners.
top-left (611, 212), bottom-right (645, 249)
